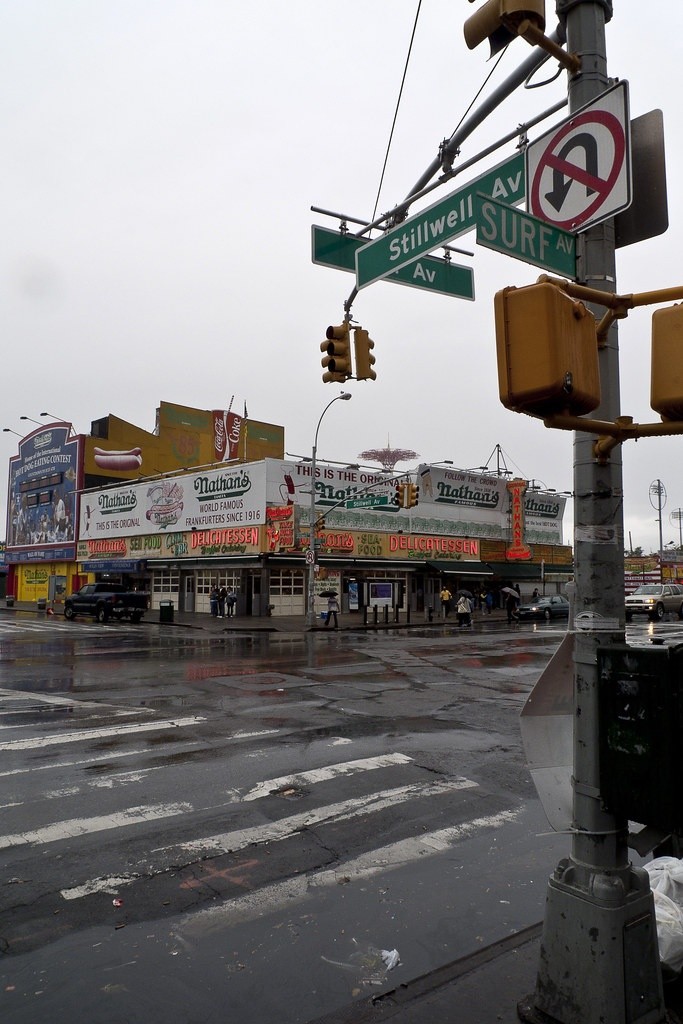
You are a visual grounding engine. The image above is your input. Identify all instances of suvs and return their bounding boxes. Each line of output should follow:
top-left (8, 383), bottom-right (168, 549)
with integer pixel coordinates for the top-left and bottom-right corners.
top-left (625, 584), bottom-right (683, 621)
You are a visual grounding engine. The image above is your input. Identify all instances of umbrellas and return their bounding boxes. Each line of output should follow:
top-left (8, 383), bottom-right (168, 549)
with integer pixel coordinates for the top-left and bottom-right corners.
top-left (319, 591), bottom-right (339, 597)
top-left (501, 587), bottom-right (519, 600)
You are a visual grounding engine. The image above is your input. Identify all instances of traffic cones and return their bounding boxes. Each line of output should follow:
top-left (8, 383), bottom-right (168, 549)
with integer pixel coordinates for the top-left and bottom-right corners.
top-left (46, 608), bottom-right (54, 615)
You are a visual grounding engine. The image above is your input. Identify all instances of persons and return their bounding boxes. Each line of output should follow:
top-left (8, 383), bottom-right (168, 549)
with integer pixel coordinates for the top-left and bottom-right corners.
top-left (455, 592), bottom-right (474, 627)
top-left (15, 489), bottom-right (73, 545)
top-left (513, 584), bottom-right (520, 596)
top-left (532, 588), bottom-right (540, 597)
top-left (479, 590), bottom-right (500, 616)
top-left (208, 583), bottom-right (237, 619)
top-left (325, 596), bottom-right (339, 628)
top-left (504, 593), bottom-right (520, 626)
top-left (439, 586), bottom-right (452, 617)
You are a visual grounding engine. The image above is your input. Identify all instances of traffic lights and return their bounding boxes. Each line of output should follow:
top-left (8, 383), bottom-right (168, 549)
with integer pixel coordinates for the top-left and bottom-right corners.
top-left (464, 0), bottom-right (520, 63)
top-left (327, 323), bottom-right (353, 376)
top-left (408, 484), bottom-right (420, 507)
top-left (494, 285), bottom-right (601, 419)
top-left (317, 512), bottom-right (325, 531)
top-left (395, 484), bottom-right (404, 507)
top-left (355, 330), bottom-right (377, 380)
top-left (320, 339), bottom-right (347, 384)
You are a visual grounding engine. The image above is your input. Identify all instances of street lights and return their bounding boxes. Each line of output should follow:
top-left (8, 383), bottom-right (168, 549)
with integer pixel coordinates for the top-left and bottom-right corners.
top-left (307, 392), bottom-right (352, 630)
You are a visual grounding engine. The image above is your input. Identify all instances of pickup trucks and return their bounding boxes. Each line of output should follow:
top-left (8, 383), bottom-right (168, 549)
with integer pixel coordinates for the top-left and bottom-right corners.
top-left (64, 582), bottom-right (152, 624)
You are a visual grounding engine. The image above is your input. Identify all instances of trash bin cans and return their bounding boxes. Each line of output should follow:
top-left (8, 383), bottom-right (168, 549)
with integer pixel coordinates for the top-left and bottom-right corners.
top-left (424, 603), bottom-right (433, 622)
top-left (37, 598), bottom-right (46, 610)
top-left (159, 599), bottom-right (174, 622)
top-left (6, 595), bottom-right (14, 606)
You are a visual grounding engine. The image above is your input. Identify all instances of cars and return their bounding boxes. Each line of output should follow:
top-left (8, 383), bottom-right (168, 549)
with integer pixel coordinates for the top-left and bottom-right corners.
top-left (514, 594), bottom-right (570, 621)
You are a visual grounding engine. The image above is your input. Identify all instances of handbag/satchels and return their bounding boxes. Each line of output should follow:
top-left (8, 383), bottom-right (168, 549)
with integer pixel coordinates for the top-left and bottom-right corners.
top-left (458, 602), bottom-right (467, 613)
top-left (230, 596), bottom-right (237, 602)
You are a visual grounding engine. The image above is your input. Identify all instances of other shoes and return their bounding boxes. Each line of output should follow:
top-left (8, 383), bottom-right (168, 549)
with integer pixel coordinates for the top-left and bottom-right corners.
top-left (515, 616), bottom-right (519, 624)
top-left (506, 622), bottom-right (512, 624)
top-left (217, 615), bottom-right (225, 618)
top-left (226, 615), bottom-right (234, 618)
